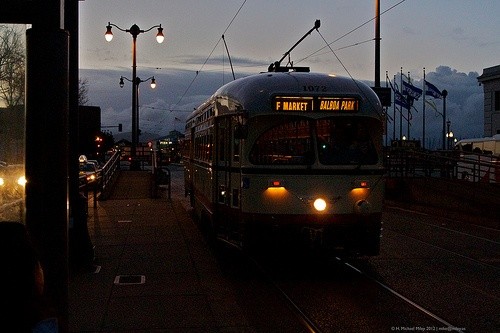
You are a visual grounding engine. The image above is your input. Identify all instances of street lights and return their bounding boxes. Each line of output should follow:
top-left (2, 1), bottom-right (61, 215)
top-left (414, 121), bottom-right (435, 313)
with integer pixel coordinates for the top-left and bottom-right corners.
top-left (120, 75), bottom-right (157, 156)
top-left (446, 118), bottom-right (451, 150)
top-left (104, 21), bottom-right (165, 172)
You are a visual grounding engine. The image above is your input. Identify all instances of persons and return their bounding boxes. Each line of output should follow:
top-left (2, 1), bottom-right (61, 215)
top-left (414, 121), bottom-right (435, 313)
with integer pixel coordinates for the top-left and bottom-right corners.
top-left (0, 222), bottom-right (58, 333)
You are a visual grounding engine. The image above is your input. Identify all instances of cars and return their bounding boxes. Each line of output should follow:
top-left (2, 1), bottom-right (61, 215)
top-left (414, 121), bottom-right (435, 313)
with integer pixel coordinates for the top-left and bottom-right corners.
top-left (0, 161), bottom-right (25, 198)
top-left (79, 142), bottom-right (175, 187)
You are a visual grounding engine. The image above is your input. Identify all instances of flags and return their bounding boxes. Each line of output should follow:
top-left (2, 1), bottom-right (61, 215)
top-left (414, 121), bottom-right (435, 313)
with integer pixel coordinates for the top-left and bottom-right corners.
top-left (395, 79), bottom-right (443, 110)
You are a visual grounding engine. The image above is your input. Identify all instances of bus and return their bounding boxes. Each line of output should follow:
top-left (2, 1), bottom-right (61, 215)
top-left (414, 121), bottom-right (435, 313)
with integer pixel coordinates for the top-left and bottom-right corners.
top-left (184, 18), bottom-right (388, 271)
